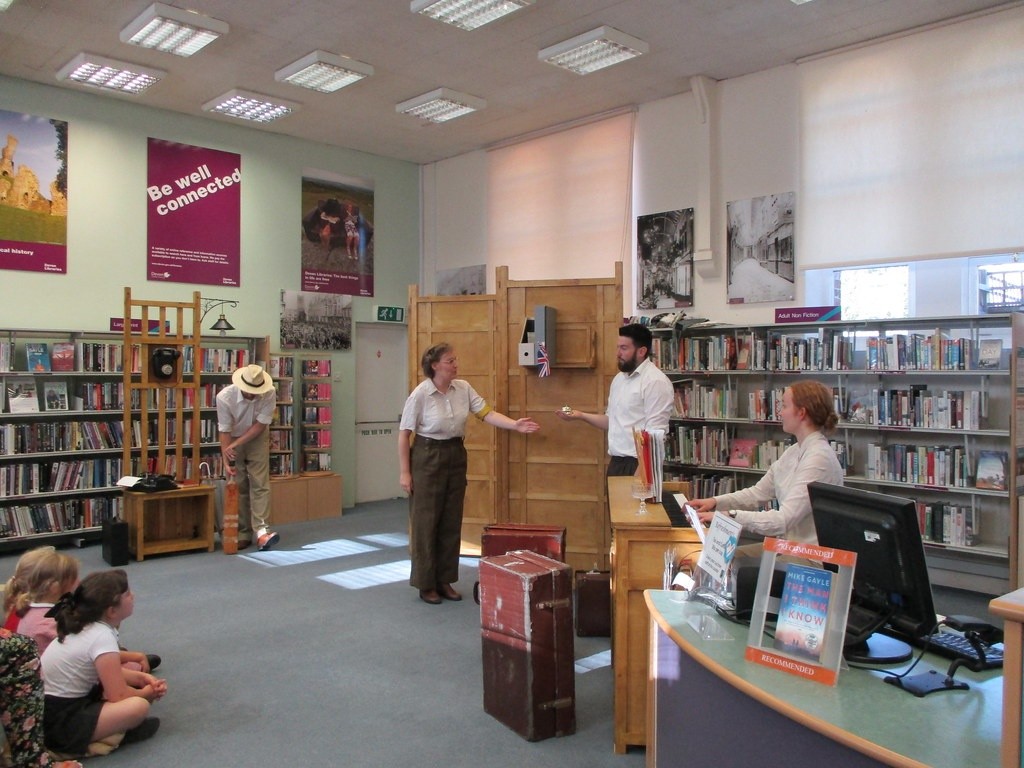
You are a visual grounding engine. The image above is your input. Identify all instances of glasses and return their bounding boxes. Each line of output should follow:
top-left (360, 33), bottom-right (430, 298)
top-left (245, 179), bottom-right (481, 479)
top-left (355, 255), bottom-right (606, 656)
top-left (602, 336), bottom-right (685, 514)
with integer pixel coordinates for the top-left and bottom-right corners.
top-left (439, 356), bottom-right (459, 365)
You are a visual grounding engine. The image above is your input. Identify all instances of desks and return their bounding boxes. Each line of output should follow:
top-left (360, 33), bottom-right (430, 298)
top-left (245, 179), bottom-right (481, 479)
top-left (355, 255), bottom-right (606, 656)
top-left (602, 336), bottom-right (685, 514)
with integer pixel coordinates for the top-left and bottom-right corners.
top-left (606, 476), bottom-right (709, 758)
top-left (120, 484), bottom-right (216, 562)
top-left (644, 586), bottom-right (1004, 768)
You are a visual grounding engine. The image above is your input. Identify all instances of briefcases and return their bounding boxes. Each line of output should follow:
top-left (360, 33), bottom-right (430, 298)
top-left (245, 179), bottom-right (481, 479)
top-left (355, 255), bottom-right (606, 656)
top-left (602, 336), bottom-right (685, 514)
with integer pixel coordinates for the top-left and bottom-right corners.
top-left (575, 568), bottom-right (611, 637)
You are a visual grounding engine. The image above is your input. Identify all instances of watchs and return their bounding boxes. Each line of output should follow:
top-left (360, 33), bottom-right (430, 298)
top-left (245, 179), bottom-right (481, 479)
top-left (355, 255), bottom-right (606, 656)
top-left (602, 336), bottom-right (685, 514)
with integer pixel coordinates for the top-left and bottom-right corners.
top-left (728, 509), bottom-right (737, 521)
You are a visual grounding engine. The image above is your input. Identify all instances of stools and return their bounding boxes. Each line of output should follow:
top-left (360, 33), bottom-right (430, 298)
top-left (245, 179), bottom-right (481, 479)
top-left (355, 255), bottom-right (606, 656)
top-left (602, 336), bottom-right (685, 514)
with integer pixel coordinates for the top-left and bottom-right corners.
top-left (575, 570), bottom-right (610, 637)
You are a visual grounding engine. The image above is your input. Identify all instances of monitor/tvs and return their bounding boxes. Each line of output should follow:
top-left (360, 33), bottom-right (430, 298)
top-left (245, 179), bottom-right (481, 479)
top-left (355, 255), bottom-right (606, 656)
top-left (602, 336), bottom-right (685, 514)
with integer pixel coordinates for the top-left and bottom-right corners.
top-left (808, 479), bottom-right (940, 660)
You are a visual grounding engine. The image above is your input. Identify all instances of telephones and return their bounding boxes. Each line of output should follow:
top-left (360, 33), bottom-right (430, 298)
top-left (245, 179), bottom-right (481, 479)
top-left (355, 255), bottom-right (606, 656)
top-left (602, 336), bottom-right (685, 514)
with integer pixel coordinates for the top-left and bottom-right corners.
top-left (945, 613), bottom-right (1004, 642)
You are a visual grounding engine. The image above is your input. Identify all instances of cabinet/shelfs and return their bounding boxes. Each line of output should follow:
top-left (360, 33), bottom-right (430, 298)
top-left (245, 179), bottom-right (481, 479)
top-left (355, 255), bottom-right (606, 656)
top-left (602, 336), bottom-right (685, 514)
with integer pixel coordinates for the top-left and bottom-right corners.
top-left (299, 351), bottom-right (334, 477)
top-left (270, 352), bottom-right (296, 480)
top-left (0, 327), bottom-right (271, 544)
top-left (268, 471), bottom-right (343, 525)
top-left (649, 310), bottom-right (1024, 597)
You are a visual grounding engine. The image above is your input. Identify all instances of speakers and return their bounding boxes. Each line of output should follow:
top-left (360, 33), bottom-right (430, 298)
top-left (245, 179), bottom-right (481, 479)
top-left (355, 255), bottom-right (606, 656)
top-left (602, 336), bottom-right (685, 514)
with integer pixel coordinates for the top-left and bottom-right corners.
top-left (102, 518), bottom-right (129, 565)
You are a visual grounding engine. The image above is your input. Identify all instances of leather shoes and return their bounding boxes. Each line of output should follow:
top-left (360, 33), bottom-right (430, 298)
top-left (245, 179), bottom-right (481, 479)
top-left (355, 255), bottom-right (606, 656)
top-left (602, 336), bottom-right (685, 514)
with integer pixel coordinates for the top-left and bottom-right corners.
top-left (238, 540), bottom-right (252, 550)
top-left (257, 531), bottom-right (280, 551)
top-left (420, 590), bottom-right (442, 604)
top-left (439, 582), bottom-right (462, 601)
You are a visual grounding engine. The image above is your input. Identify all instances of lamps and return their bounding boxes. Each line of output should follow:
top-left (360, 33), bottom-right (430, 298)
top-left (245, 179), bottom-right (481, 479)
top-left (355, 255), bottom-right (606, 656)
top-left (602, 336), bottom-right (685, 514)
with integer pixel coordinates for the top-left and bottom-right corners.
top-left (200, 297), bottom-right (240, 338)
top-left (409, 0), bottom-right (531, 33)
top-left (538, 25), bottom-right (650, 76)
top-left (55, 51), bottom-right (168, 99)
top-left (201, 88), bottom-right (304, 127)
top-left (119, 3), bottom-right (229, 59)
top-left (274, 49), bottom-right (374, 94)
top-left (395, 87), bottom-right (487, 125)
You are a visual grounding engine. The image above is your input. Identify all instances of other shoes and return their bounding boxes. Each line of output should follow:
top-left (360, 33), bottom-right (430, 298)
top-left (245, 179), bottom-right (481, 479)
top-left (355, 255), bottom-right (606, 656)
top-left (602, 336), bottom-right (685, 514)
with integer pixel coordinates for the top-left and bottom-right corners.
top-left (119, 716), bottom-right (160, 746)
top-left (145, 654), bottom-right (161, 670)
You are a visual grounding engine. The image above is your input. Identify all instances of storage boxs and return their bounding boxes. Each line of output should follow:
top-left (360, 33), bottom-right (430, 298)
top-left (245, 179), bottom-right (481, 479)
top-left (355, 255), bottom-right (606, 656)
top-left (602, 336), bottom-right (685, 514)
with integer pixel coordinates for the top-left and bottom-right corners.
top-left (481, 522), bottom-right (567, 565)
top-left (478, 548), bottom-right (579, 741)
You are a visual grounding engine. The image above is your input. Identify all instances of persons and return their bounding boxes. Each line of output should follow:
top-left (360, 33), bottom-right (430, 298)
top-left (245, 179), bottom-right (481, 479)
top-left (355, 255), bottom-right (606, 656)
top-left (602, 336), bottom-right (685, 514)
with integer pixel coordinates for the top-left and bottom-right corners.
top-left (634, 330), bottom-right (1014, 551)
top-left (0, 545), bottom-right (167, 768)
top-left (216, 364), bottom-right (280, 552)
top-left (396, 342), bottom-right (541, 604)
top-left (681, 380), bottom-right (844, 602)
top-left (554, 324), bottom-right (674, 541)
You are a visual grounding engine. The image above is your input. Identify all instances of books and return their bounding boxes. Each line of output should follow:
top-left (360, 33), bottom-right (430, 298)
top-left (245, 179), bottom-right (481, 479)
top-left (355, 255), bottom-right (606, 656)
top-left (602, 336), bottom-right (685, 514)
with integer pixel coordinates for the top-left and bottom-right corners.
top-left (0, 337), bottom-right (333, 539)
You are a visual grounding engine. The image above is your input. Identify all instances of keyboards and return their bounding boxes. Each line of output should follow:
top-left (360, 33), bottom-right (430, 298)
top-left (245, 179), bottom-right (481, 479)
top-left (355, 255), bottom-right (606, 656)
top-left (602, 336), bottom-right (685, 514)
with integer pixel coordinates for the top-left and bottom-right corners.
top-left (917, 631), bottom-right (1004, 671)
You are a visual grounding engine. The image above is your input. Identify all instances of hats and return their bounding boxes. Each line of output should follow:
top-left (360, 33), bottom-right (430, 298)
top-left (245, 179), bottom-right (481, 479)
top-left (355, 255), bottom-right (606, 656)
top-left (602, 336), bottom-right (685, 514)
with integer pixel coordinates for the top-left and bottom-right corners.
top-left (232, 364), bottom-right (272, 394)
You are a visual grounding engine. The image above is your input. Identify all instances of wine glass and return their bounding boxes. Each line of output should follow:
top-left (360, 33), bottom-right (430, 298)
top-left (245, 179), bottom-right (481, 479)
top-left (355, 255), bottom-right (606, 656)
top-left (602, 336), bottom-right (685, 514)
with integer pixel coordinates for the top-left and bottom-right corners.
top-left (630, 483), bottom-right (655, 517)
